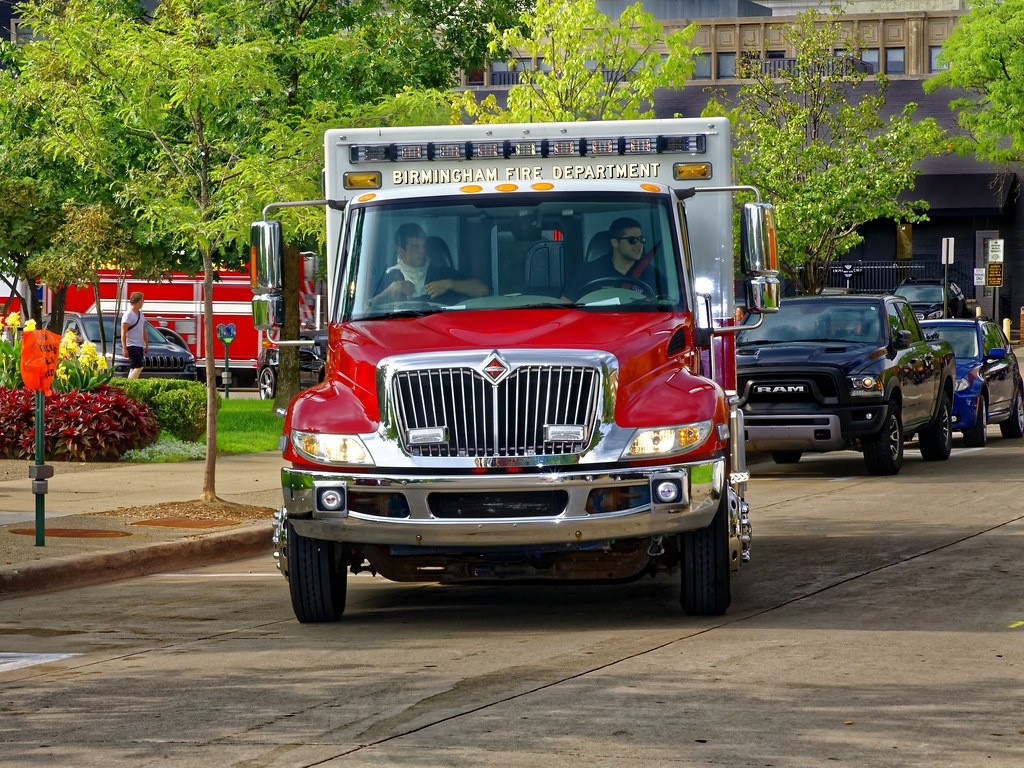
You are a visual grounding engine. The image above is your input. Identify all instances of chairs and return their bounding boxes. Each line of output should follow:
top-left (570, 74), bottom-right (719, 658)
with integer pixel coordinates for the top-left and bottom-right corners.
top-left (425, 236), bottom-right (455, 270)
top-left (585, 231), bottom-right (646, 260)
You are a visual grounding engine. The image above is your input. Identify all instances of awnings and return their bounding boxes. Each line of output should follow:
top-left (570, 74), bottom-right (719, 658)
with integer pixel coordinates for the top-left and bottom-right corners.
top-left (873, 172), bottom-right (1016, 208)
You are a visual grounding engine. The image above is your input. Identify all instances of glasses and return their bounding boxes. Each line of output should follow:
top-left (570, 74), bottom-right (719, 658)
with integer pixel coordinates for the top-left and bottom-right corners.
top-left (615, 236), bottom-right (647, 245)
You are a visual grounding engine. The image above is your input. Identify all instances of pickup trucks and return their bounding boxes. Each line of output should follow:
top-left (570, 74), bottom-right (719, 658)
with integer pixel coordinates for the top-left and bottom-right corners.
top-left (735, 295), bottom-right (957, 474)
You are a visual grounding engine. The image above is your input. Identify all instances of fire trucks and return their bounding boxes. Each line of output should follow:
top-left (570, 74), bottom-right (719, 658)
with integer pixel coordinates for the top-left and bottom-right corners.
top-left (1, 254), bottom-right (320, 385)
top-left (249, 118), bottom-right (780, 629)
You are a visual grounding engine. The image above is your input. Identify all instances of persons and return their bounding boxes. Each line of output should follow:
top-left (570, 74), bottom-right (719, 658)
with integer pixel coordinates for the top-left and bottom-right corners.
top-left (366, 223), bottom-right (490, 306)
top-left (807, 309), bottom-right (875, 342)
top-left (561, 217), bottom-right (668, 303)
top-left (121, 292), bottom-right (149, 379)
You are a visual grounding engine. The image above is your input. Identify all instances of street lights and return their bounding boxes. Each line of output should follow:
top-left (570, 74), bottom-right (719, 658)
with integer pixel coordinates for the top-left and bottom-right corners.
top-left (844, 264), bottom-right (854, 288)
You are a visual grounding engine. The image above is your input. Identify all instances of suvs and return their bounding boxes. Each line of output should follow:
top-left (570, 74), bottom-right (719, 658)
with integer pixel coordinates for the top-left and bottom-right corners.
top-left (892, 278), bottom-right (968, 321)
top-left (40, 311), bottom-right (198, 380)
top-left (918, 314), bottom-right (1023, 445)
top-left (801, 288), bottom-right (868, 337)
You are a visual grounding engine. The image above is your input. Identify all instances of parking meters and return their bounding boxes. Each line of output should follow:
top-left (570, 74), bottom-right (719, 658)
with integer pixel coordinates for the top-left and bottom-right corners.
top-left (215, 322), bottom-right (237, 398)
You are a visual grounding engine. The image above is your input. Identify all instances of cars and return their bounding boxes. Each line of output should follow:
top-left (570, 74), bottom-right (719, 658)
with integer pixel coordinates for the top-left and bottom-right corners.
top-left (257, 328), bottom-right (328, 401)
